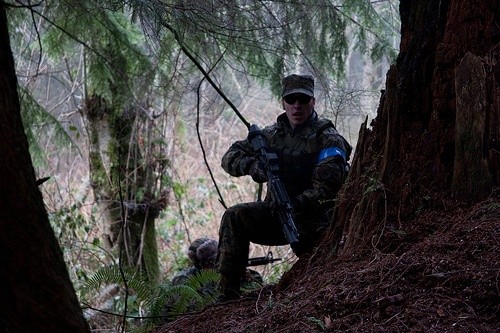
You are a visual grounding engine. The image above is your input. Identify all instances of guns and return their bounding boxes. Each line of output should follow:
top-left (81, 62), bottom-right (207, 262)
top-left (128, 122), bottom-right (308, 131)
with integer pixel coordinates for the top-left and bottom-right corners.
top-left (247, 124), bottom-right (300, 247)
top-left (246, 251), bottom-right (282, 267)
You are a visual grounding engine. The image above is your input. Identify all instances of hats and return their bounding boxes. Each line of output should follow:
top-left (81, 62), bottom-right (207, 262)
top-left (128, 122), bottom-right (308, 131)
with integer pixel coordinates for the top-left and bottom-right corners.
top-left (282, 74), bottom-right (314, 97)
top-left (188, 238), bottom-right (218, 264)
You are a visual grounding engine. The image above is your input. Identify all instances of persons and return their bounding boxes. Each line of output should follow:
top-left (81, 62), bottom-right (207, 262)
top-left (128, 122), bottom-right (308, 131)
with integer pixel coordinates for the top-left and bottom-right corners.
top-left (172, 237), bottom-right (264, 299)
top-left (217, 74), bottom-right (354, 301)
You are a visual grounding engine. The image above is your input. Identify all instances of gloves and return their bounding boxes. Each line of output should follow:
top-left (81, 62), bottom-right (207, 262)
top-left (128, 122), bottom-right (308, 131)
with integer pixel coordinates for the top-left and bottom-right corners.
top-left (270, 199), bottom-right (293, 217)
top-left (248, 160), bottom-right (269, 183)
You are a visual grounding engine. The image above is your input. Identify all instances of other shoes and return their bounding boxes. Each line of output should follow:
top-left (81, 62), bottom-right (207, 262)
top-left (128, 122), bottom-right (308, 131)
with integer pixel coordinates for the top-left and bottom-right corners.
top-left (216, 281), bottom-right (240, 304)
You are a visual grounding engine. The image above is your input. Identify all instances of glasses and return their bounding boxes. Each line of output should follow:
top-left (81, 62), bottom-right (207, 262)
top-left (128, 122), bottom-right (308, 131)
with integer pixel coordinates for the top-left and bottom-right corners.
top-left (283, 94), bottom-right (312, 104)
